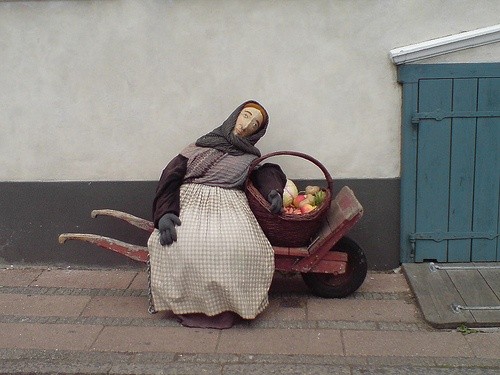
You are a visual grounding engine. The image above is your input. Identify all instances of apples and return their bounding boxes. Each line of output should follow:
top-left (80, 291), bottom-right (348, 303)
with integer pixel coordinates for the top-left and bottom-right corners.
top-left (302, 204), bottom-right (312, 214)
top-left (293, 195), bottom-right (305, 208)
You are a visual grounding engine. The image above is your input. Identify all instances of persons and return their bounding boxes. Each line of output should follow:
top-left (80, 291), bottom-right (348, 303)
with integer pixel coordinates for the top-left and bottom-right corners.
top-left (144, 99), bottom-right (287, 331)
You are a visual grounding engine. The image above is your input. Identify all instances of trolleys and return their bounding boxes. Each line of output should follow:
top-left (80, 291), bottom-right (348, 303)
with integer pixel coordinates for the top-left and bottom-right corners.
top-left (59, 186), bottom-right (367, 298)
